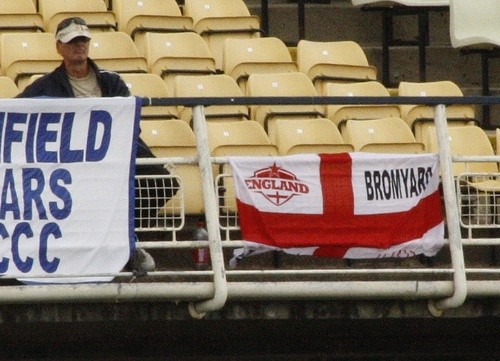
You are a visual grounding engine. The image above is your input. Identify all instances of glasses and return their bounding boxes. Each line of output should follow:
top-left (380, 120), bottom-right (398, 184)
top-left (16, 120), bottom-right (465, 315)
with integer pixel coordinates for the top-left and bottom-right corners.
top-left (56, 17), bottom-right (86, 34)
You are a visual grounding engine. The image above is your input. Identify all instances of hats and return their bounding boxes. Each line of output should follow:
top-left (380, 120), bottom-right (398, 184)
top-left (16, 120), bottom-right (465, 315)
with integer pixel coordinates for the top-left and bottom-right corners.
top-left (55, 16), bottom-right (92, 43)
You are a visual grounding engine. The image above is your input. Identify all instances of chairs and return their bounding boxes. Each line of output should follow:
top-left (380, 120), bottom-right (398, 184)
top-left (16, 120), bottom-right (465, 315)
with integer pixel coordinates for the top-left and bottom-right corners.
top-left (1, 0), bottom-right (500, 213)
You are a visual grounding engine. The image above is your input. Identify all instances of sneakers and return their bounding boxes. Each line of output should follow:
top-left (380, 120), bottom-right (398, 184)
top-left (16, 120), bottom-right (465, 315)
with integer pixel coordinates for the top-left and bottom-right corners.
top-left (127, 234), bottom-right (156, 270)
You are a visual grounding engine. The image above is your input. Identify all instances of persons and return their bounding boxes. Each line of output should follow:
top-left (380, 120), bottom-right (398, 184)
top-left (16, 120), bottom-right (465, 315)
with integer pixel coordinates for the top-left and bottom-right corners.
top-left (13, 17), bottom-right (180, 271)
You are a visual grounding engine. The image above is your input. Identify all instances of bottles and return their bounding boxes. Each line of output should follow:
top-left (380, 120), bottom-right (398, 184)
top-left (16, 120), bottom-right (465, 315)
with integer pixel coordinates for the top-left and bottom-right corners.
top-left (193, 222), bottom-right (209, 270)
